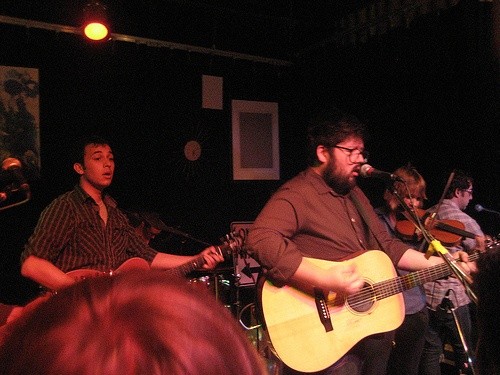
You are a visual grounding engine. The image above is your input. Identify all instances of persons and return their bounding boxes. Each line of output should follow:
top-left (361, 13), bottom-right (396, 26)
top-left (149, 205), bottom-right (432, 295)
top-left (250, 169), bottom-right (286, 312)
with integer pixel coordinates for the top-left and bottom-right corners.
top-left (20, 136), bottom-right (224, 293)
top-left (0, 269), bottom-right (270, 375)
top-left (247, 115), bottom-right (490, 375)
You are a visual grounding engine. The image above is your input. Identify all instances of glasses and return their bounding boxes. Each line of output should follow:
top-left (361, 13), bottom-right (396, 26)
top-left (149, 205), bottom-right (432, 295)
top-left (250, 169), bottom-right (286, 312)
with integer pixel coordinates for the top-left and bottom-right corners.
top-left (322, 142), bottom-right (369, 164)
top-left (460, 187), bottom-right (474, 196)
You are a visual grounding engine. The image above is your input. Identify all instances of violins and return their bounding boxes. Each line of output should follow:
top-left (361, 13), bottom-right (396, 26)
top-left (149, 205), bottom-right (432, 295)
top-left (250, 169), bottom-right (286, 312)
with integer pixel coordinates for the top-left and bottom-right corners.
top-left (395, 208), bottom-right (500, 247)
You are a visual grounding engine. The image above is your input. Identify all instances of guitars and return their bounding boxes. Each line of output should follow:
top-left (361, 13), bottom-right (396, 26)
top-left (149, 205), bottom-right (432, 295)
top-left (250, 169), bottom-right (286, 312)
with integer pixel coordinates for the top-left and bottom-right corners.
top-left (255, 240), bottom-right (500, 373)
top-left (40, 226), bottom-right (245, 291)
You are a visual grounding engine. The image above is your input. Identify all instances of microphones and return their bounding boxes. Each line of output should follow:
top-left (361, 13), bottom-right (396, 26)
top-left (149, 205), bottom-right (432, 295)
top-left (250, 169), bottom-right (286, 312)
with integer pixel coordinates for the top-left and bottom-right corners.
top-left (360, 164), bottom-right (404, 182)
top-left (475, 203), bottom-right (500, 215)
top-left (2, 157), bottom-right (31, 196)
top-left (438, 291), bottom-right (450, 316)
